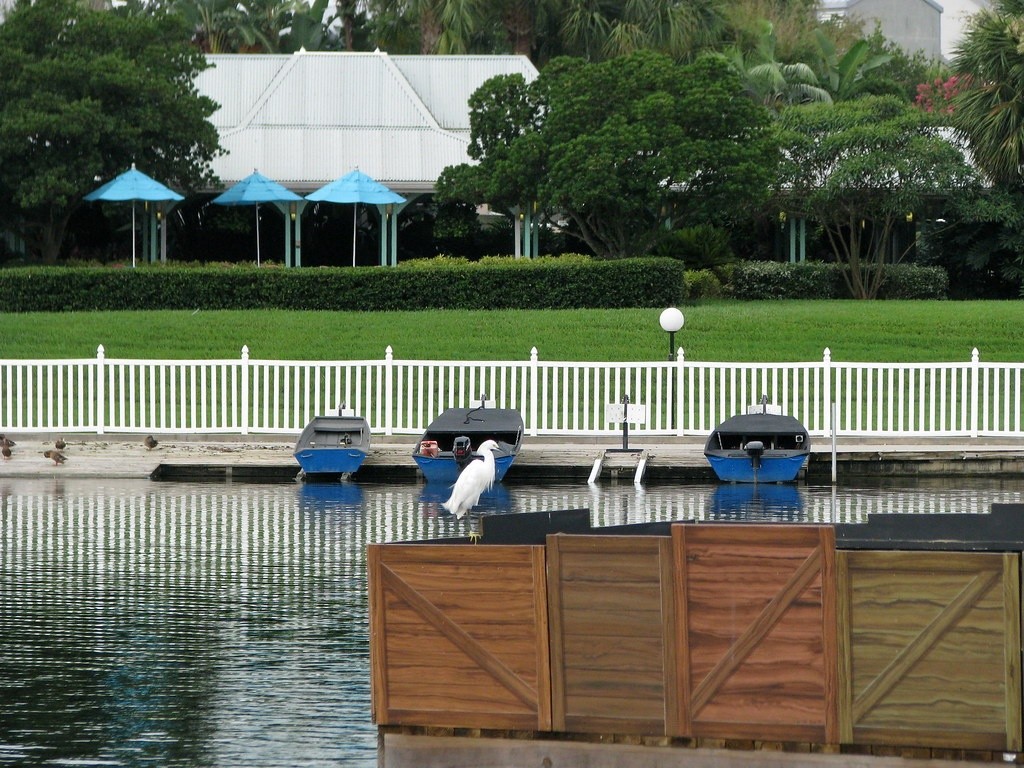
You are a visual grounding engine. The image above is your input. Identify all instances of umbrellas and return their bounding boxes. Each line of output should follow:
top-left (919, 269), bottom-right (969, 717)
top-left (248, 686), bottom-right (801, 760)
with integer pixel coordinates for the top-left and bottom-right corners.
top-left (304, 165), bottom-right (407, 266)
top-left (83, 163), bottom-right (185, 266)
top-left (212, 168), bottom-right (303, 267)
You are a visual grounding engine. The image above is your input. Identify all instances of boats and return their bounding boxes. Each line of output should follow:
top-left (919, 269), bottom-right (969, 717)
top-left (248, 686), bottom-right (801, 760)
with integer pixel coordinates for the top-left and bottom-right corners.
top-left (293, 416), bottom-right (372, 475)
top-left (704, 413), bottom-right (811, 484)
top-left (412, 407), bottom-right (524, 484)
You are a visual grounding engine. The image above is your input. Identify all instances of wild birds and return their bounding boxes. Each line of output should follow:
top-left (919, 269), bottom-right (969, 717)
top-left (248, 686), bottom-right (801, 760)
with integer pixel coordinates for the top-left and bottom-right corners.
top-left (0, 433), bottom-right (17, 460)
top-left (44, 450), bottom-right (69, 465)
top-left (55, 435), bottom-right (66, 452)
top-left (439, 440), bottom-right (502, 523)
top-left (144, 435), bottom-right (158, 451)
